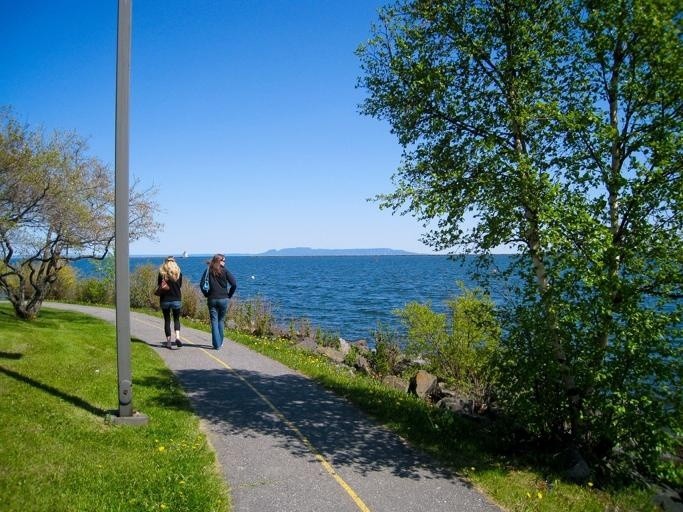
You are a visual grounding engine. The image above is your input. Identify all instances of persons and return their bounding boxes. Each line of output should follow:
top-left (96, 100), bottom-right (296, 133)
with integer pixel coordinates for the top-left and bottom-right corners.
top-left (199, 254), bottom-right (237, 350)
top-left (157, 256), bottom-right (184, 349)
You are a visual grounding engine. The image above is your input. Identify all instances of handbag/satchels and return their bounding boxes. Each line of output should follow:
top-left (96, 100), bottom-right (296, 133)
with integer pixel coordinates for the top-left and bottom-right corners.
top-left (154, 279), bottom-right (170, 296)
top-left (202, 279), bottom-right (210, 293)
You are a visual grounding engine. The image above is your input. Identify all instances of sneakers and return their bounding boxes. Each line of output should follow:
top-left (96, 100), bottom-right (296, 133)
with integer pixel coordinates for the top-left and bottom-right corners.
top-left (176, 339), bottom-right (182, 347)
top-left (167, 342), bottom-right (172, 347)
